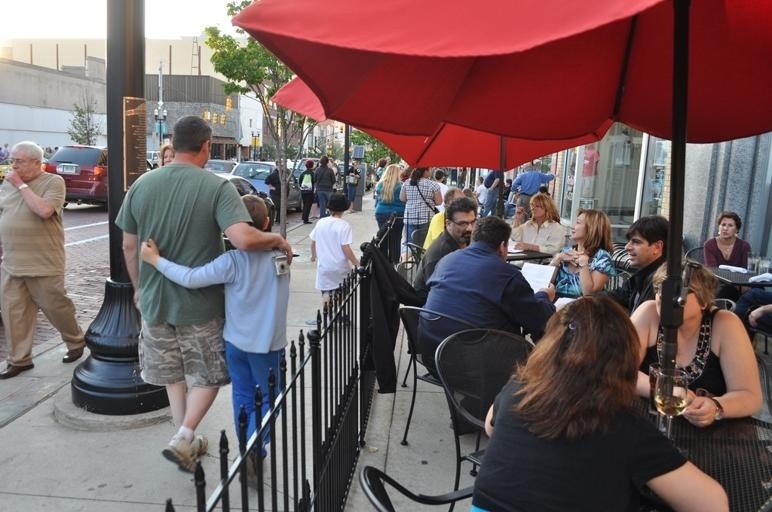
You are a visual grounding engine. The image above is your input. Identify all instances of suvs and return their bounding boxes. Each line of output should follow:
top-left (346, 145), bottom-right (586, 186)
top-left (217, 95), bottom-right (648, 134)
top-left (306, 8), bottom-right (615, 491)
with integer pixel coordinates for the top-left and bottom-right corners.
top-left (40, 142), bottom-right (153, 214)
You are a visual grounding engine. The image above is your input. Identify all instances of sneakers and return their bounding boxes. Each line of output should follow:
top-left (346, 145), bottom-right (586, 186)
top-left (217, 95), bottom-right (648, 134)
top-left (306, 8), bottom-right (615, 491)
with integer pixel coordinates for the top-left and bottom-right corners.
top-left (162, 434), bottom-right (208, 473)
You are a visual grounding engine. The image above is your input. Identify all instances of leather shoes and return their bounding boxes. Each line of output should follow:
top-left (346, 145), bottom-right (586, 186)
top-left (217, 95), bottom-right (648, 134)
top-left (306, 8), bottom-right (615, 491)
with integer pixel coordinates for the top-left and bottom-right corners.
top-left (63, 347), bottom-right (83, 362)
top-left (0, 364), bottom-right (34, 378)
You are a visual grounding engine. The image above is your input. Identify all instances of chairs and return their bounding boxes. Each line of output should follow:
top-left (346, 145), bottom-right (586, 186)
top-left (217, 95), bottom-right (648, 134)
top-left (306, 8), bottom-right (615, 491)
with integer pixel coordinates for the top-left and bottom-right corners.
top-left (410, 228), bottom-right (429, 271)
top-left (435, 329), bottom-right (535, 512)
top-left (400, 304), bottom-right (482, 476)
top-left (601, 242), bottom-right (638, 292)
top-left (360, 466), bottom-right (474, 512)
top-left (685, 247), bottom-right (771, 414)
top-left (397, 262), bottom-right (423, 388)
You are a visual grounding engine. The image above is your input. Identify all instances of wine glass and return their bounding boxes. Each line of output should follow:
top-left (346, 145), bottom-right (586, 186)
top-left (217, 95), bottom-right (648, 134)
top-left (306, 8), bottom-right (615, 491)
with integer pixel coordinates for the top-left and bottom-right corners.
top-left (655, 369), bottom-right (689, 447)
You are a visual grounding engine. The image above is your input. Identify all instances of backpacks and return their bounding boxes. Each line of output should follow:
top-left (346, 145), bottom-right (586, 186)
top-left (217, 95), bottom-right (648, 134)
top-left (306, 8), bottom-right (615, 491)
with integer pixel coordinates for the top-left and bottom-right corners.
top-left (299, 174), bottom-right (312, 191)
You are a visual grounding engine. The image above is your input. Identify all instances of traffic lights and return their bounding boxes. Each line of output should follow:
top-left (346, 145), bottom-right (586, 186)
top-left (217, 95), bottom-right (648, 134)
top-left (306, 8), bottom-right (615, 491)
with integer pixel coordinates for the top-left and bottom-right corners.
top-left (340, 126), bottom-right (343, 133)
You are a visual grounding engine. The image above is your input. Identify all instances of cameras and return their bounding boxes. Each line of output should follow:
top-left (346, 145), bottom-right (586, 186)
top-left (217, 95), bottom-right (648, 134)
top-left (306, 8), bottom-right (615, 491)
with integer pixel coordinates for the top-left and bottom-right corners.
top-left (274, 255), bottom-right (288, 276)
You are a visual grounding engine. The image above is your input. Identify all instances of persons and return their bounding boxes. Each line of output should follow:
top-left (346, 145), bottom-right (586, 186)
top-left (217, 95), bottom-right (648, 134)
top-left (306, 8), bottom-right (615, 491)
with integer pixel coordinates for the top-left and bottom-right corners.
top-left (0, 143), bottom-right (59, 165)
top-left (0, 140), bottom-right (86, 379)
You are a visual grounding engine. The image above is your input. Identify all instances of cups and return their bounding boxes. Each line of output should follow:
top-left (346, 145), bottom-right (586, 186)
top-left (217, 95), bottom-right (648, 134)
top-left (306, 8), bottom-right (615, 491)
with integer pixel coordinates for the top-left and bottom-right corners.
top-left (648, 364), bottom-right (662, 416)
top-left (746, 251), bottom-right (770, 276)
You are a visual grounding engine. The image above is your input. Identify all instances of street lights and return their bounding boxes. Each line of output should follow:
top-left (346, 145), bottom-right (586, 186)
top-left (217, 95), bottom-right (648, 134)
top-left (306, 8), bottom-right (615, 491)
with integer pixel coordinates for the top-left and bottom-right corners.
top-left (251, 129), bottom-right (262, 162)
top-left (154, 108), bottom-right (168, 144)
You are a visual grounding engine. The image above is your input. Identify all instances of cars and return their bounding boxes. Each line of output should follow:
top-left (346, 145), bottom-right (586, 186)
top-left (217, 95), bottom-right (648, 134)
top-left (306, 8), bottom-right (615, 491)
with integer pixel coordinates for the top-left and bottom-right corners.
top-left (216, 172), bottom-right (276, 252)
top-left (146, 150), bottom-right (377, 216)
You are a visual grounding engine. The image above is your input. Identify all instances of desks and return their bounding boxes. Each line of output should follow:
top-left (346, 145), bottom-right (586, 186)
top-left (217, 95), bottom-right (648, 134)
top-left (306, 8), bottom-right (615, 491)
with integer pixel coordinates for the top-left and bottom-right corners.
top-left (647, 416), bottom-right (771, 512)
top-left (505, 250), bottom-right (552, 271)
